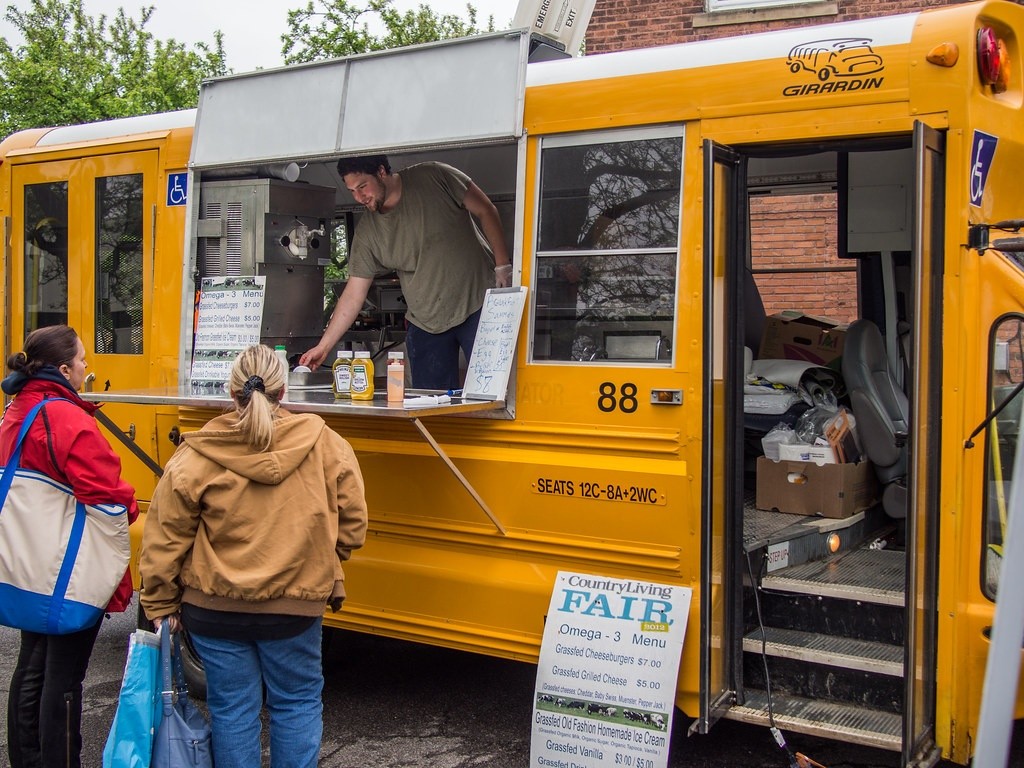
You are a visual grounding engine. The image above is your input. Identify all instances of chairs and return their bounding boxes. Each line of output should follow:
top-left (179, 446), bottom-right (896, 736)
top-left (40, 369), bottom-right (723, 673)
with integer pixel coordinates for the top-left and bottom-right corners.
top-left (839, 318), bottom-right (1011, 551)
top-left (742, 268), bottom-right (814, 436)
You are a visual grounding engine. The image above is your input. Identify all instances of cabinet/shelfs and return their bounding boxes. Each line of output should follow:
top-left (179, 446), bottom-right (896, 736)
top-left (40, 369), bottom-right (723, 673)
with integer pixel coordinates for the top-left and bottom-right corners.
top-left (380, 289), bottom-right (410, 329)
top-left (350, 339), bottom-right (410, 378)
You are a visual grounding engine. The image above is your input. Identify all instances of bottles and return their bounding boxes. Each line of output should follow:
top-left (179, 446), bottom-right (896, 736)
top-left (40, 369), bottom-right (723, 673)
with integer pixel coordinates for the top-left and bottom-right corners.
top-left (274, 345), bottom-right (290, 393)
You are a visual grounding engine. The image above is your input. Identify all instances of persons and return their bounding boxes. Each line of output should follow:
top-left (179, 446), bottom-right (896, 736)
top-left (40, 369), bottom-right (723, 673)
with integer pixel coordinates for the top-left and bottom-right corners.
top-left (299, 154), bottom-right (513, 390)
top-left (0, 325), bottom-right (138, 768)
top-left (139, 345), bottom-right (368, 768)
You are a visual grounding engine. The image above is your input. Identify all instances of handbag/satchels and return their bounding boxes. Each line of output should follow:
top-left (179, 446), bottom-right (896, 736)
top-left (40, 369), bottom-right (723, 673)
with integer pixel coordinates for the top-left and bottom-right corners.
top-left (102, 619), bottom-right (212, 768)
top-left (0, 398), bottom-right (131, 634)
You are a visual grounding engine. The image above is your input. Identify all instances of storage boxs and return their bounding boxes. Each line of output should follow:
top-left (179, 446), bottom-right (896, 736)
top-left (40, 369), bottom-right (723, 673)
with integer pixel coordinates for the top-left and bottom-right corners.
top-left (758, 308), bottom-right (848, 373)
top-left (756, 452), bottom-right (872, 521)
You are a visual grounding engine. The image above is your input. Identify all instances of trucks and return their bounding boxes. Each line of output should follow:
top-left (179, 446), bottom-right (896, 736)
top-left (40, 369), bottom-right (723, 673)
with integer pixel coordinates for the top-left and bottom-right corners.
top-left (0, 1), bottom-right (1024, 768)
